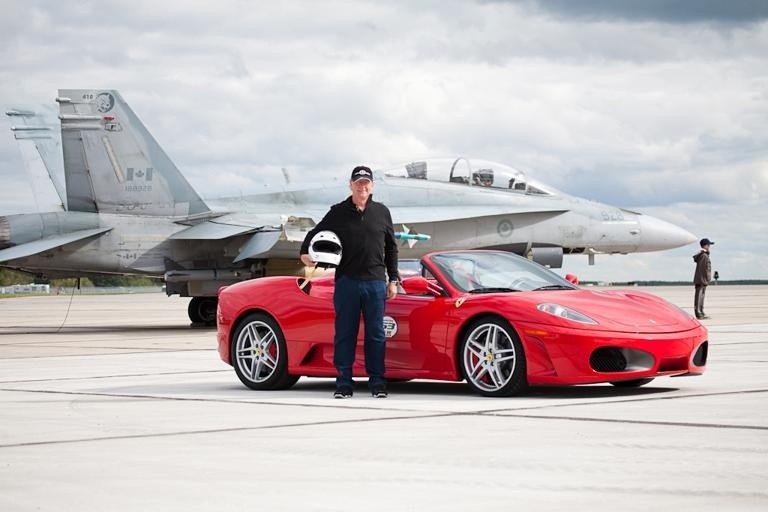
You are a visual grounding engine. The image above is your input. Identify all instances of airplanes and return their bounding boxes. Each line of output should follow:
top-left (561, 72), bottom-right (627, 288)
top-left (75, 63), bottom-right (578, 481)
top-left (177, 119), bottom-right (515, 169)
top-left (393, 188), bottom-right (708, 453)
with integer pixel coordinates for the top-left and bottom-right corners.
top-left (0, 84), bottom-right (699, 334)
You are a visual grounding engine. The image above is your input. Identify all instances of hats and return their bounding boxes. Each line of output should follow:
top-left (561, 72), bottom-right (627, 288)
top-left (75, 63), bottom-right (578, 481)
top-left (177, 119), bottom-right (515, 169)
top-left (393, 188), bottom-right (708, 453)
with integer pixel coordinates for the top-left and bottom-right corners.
top-left (351, 166), bottom-right (374, 184)
top-left (699, 238), bottom-right (714, 247)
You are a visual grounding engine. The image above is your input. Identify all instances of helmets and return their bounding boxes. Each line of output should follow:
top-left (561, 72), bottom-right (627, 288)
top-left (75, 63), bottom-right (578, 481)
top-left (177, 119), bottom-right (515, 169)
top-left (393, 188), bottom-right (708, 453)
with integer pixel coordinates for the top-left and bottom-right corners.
top-left (308, 231), bottom-right (344, 270)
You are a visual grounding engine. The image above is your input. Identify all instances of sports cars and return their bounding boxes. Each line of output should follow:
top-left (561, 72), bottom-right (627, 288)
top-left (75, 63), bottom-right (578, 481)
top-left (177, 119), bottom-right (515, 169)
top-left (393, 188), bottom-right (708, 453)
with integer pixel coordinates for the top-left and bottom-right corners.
top-left (215, 248), bottom-right (711, 398)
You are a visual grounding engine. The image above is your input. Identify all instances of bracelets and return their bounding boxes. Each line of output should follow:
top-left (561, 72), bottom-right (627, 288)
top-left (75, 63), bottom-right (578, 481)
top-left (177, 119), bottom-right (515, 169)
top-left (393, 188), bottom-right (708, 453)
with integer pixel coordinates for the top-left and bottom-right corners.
top-left (389, 280), bottom-right (397, 286)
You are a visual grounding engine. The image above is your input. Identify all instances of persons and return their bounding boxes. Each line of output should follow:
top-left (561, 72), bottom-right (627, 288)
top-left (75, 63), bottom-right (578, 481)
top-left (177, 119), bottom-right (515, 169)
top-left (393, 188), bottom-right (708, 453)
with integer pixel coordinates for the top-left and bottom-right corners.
top-left (692, 238), bottom-right (714, 320)
top-left (299, 166), bottom-right (398, 399)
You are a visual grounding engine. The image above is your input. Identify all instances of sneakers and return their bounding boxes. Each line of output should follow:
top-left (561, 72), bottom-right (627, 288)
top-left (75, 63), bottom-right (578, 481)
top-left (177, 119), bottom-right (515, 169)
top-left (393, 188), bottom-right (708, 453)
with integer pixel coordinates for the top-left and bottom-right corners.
top-left (334, 385), bottom-right (354, 399)
top-left (371, 384), bottom-right (387, 398)
